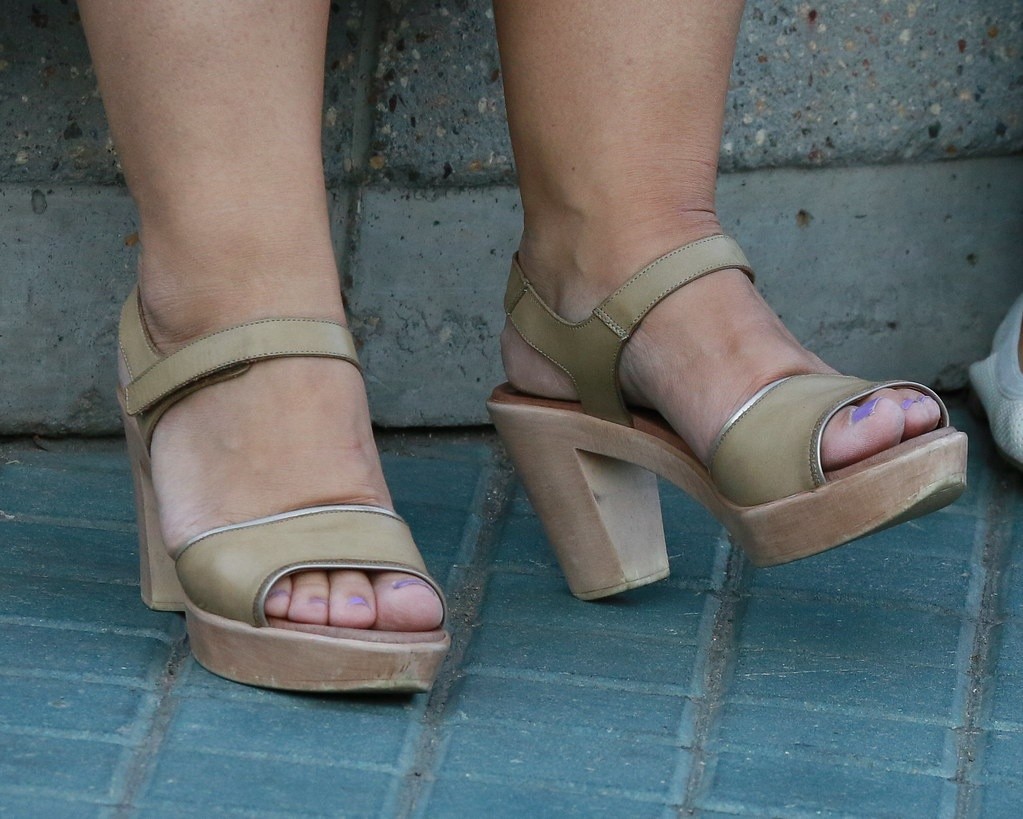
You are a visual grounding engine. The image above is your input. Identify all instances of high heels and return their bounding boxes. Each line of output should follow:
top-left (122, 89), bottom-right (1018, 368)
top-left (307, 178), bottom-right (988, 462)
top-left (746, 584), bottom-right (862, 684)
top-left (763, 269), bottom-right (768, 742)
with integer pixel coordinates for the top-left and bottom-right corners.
top-left (486, 236), bottom-right (968, 599)
top-left (115, 283), bottom-right (451, 692)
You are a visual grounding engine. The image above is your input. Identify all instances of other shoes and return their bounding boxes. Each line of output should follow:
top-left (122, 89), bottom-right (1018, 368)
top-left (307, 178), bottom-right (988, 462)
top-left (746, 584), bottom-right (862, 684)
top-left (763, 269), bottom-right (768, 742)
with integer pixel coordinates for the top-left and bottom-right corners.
top-left (968, 295), bottom-right (1023, 472)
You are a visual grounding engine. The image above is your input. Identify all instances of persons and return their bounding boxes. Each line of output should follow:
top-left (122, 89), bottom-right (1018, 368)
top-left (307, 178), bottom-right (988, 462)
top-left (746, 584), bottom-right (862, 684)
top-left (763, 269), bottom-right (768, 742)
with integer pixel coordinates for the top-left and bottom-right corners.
top-left (74, 0), bottom-right (967, 693)
